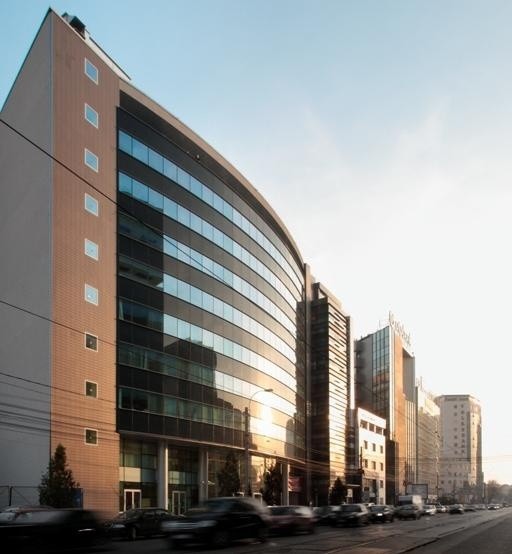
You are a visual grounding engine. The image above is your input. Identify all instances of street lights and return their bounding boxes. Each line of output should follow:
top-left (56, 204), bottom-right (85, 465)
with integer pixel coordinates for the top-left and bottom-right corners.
top-left (243, 383), bottom-right (275, 501)
